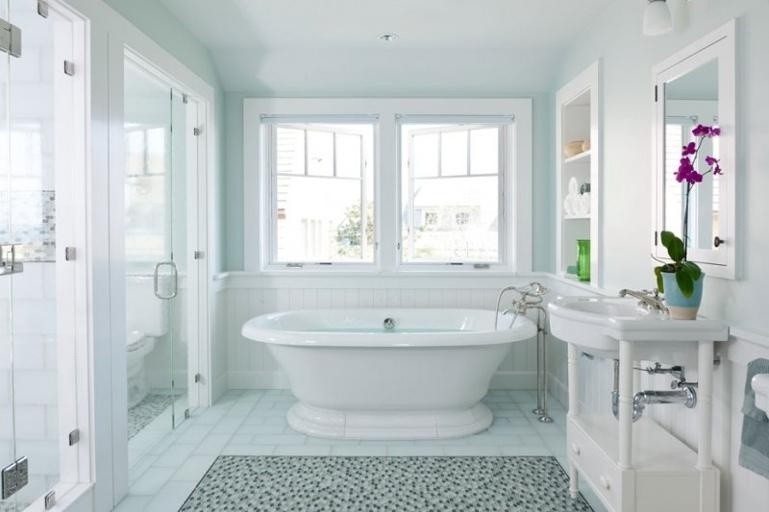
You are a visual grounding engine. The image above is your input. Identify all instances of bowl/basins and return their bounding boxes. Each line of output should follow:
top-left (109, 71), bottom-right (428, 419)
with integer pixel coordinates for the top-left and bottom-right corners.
top-left (563, 138), bottom-right (588, 157)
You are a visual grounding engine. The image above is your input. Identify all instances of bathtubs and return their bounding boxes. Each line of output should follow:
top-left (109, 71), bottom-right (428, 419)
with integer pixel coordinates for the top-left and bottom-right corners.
top-left (239, 306), bottom-right (538, 412)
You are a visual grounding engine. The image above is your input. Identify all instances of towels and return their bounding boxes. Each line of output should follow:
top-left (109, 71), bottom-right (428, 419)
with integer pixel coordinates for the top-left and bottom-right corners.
top-left (564, 177), bottom-right (589, 217)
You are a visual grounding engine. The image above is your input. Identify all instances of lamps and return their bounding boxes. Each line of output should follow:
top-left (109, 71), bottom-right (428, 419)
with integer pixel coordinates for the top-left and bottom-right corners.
top-left (639, 0), bottom-right (677, 34)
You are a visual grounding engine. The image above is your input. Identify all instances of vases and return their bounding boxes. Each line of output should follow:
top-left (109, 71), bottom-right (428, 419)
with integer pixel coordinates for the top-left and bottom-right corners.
top-left (575, 239), bottom-right (589, 281)
top-left (659, 270), bottom-right (707, 321)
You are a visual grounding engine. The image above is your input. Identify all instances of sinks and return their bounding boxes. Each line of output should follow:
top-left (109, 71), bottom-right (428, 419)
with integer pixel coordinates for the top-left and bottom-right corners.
top-left (547, 295), bottom-right (729, 369)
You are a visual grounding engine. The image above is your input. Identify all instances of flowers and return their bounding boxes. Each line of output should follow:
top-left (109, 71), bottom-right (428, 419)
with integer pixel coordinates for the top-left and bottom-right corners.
top-left (654, 124), bottom-right (723, 297)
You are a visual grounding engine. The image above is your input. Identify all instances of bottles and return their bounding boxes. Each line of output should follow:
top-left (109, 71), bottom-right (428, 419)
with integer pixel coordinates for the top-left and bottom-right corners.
top-left (582, 182), bottom-right (590, 213)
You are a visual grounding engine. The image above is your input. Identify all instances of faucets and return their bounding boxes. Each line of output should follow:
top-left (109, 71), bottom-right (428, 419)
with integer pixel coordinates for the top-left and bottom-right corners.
top-left (617, 289), bottom-right (665, 315)
top-left (502, 308), bottom-right (525, 316)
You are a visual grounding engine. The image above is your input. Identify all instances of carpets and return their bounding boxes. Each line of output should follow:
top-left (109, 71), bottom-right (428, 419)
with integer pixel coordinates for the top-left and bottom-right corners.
top-left (179, 454), bottom-right (595, 511)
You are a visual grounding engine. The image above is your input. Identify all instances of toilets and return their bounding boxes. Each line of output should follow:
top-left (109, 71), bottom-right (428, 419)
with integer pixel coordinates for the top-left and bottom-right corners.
top-left (126, 273), bottom-right (170, 410)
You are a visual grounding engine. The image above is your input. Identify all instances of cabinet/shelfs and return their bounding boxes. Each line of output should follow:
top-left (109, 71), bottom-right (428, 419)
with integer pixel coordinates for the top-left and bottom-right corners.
top-left (562, 148), bottom-right (590, 289)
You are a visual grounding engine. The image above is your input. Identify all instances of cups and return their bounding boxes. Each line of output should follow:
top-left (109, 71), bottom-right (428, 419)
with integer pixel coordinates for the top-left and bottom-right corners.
top-left (576, 238), bottom-right (591, 281)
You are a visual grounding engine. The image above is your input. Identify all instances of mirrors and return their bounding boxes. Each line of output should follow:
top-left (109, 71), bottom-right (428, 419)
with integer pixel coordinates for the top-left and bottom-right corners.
top-left (656, 36), bottom-right (723, 251)
